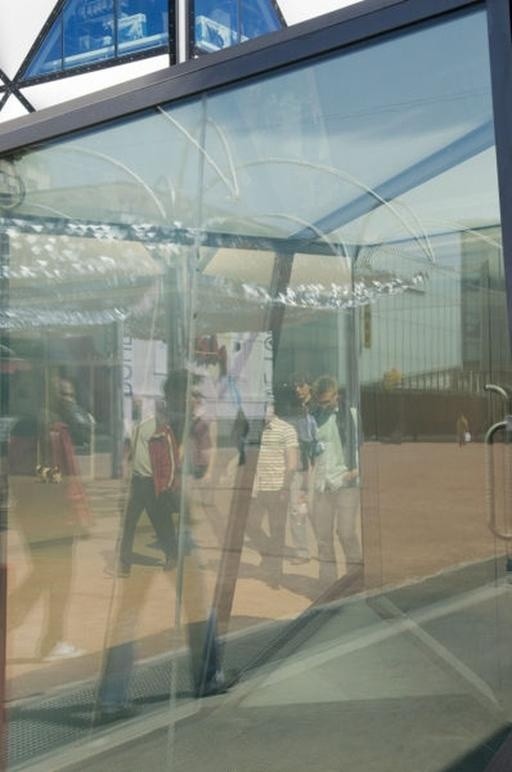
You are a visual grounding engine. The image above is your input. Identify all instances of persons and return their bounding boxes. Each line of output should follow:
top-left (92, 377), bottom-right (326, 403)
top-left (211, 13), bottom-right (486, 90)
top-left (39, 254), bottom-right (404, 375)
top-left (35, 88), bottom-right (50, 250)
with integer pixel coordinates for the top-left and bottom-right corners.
top-left (8, 364), bottom-right (370, 598)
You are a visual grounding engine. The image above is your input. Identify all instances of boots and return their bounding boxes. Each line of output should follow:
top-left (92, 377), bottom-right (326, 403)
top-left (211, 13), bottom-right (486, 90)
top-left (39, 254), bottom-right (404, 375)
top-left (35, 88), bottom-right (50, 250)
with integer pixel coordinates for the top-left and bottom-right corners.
top-left (184, 614), bottom-right (244, 698)
top-left (93, 639), bottom-right (145, 725)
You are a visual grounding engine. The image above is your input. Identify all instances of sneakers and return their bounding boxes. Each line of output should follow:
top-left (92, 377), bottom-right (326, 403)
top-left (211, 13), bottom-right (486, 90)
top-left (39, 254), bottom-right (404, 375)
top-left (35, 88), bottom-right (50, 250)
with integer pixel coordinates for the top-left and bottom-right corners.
top-left (291, 550), bottom-right (313, 565)
top-left (37, 640), bottom-right (91, 662)
top-left (103, 559), bottom-right (130, 579)
top-left (162, 555), bottom-right (177, 572)
top-left (260, 565), bottom-right (284, 591)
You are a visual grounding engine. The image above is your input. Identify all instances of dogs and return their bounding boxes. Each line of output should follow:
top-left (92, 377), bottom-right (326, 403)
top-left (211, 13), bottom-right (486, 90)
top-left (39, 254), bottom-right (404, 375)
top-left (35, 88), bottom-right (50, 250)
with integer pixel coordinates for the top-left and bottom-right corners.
top-left (34, 464), bottom-right (61, 484)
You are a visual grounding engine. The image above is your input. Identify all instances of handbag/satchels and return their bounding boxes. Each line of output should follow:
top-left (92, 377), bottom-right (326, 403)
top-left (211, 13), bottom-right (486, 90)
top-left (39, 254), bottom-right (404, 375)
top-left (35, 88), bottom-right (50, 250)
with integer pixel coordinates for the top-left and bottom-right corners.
top-left (464, 432), bottom-right (472, 443)
